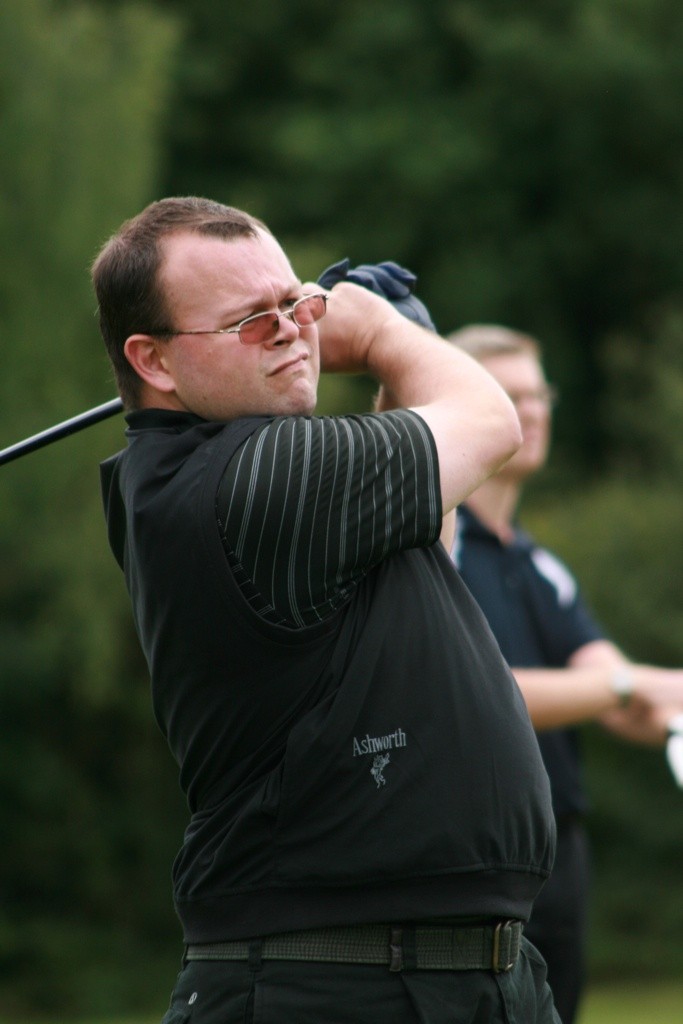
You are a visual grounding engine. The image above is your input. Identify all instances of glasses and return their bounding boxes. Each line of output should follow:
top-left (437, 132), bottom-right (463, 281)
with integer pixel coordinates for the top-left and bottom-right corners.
top-left (144, 293), bottom-right (328, 345)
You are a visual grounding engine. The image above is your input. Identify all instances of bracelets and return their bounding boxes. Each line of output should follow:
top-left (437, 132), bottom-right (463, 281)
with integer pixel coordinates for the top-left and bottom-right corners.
top-left (607, 658), bottom-right (637, 708)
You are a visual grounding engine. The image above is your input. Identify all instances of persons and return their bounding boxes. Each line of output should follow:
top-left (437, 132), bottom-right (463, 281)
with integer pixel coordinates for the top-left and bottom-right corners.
top-left (421, 321), bottom-right (683, 1023)
top-left (91, 194), bottom-right (560, 1024)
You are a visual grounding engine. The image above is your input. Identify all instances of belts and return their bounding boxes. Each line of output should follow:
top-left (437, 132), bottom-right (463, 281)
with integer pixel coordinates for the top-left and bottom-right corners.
top-left (186, 919), bottom-right (525, 977)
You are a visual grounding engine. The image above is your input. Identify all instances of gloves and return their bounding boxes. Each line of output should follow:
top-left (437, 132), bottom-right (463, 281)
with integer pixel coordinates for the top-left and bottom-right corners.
top-left (316, 256), bottom-right (433, 332)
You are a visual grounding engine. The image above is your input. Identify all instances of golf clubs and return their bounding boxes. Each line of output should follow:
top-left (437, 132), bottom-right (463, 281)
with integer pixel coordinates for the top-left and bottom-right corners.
top-left (0, 395), bottom-right (124, 466)
